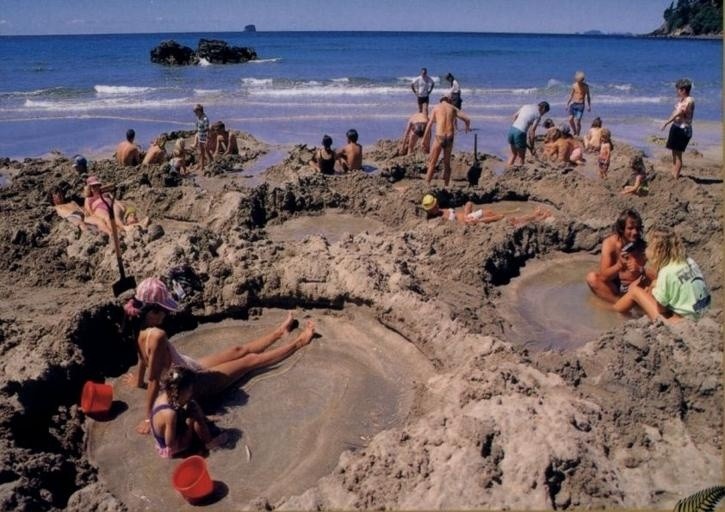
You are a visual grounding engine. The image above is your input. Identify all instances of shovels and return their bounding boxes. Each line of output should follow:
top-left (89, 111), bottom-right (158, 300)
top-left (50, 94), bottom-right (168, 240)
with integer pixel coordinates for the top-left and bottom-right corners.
top-left (468, 128), bottom-right (481, 187)
top-left (98, 183), bottom-right (137, 297)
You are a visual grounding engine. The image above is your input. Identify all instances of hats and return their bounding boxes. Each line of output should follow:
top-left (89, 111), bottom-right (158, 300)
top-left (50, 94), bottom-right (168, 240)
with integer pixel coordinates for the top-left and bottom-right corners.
top-left (422, 194), bottom-right (437, 210)
top-left (87, 177), bottom-right (101, 186)
top-left (124, 207), bottom-right (136, 223)
top-left (135, 278), bottom-right (178, 310)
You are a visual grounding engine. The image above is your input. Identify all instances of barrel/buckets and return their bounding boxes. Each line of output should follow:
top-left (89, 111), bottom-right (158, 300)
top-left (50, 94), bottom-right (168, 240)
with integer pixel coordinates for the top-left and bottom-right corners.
top-left (172, 455), bottom-right (213, 504)
top-left (80, 381), bottom-right (112, 412)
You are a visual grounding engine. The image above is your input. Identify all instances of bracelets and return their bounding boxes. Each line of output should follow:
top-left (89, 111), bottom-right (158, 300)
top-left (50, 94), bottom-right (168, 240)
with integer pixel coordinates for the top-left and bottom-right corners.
top-left (145, 419), bottom-right (152, 423)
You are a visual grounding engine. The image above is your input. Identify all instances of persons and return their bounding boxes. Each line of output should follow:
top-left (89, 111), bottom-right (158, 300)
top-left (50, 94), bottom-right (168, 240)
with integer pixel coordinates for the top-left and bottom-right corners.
top-left (123, 274), bottom-right (316, 435)
top-left (507, 71), bottom-right (649, 197)
top-left (661, 78), bottom-right (695, 179)
top-left (308, 128), bottom-right (363, 175)
top-left (585, 207), bottom-right (712, 324)
top-left (41, 104), bottom-right (239, 246)
top-left (149, 369), bottom-right (229, 458)
top-left (398, 67), bottom-right (529, 224)
